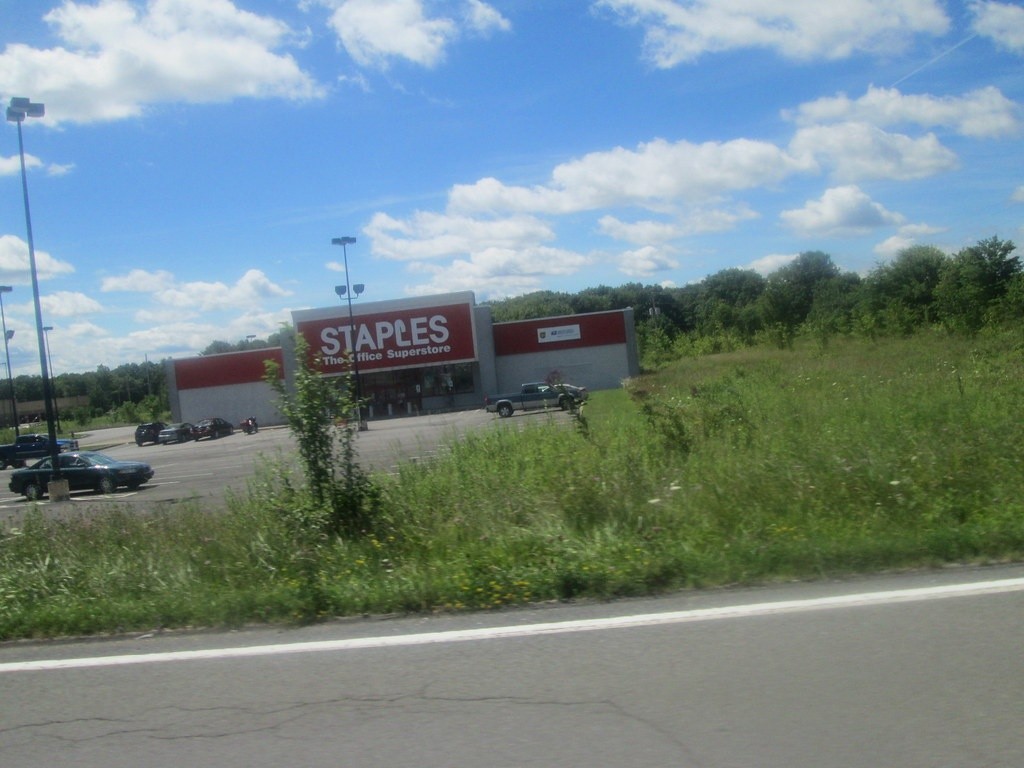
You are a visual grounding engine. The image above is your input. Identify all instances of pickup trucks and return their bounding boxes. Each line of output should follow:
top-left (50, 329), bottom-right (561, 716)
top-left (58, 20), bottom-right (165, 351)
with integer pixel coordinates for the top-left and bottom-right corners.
top-left (0, 433), bottom-right (80, 471)
top-left (484, 380), bottom-right (590, 418)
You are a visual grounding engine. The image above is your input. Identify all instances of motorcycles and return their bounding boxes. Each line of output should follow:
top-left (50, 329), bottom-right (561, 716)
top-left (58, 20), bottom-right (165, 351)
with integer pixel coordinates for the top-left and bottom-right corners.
top-left (239, 415), bottom-right (259, 434)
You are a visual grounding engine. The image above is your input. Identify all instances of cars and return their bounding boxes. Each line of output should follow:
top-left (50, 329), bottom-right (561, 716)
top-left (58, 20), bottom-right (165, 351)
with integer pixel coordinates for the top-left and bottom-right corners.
top-left (157, 422), bottom-right (195, 445)
top-left (8, 450), bottom-right (155, 501)
top-left (189, 417), bottom-right (234, 441)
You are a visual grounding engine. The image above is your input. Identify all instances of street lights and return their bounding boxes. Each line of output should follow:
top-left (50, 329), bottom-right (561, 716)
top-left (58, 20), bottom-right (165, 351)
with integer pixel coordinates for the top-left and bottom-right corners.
top-left (0, 285), bottom-right (20, 441)
top-left (4, 93), bottom-right (63, 484)
top-left (331, 235), bottom-right (367, 432)
top-left (43, 325), bottom-right (63, 434)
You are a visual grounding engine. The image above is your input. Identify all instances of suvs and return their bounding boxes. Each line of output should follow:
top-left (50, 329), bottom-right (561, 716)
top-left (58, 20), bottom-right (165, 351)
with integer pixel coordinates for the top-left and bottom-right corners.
top-left (134, 421), bottom-right (170, 447)
